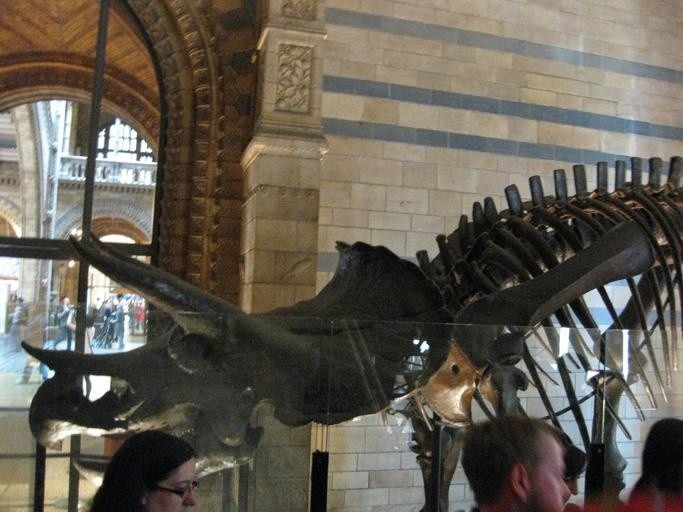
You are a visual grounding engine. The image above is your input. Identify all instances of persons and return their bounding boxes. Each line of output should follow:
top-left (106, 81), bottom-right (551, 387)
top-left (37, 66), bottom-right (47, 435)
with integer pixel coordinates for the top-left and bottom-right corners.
top-left (461, 414), bottom-right (572, 512)
top-left (628, 416), bottom-right (683, 511)
top-left (86, 430), bottom-right (200, 511)
top-left (3, 291), bottom-right (146, 384)
top-left (558, 431), bottom-right (586, 511)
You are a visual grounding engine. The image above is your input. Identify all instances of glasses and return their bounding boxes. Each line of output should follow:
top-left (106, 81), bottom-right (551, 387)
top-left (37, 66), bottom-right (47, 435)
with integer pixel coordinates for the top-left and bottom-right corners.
top-left (157, 481), bottom-right (198, 497)
